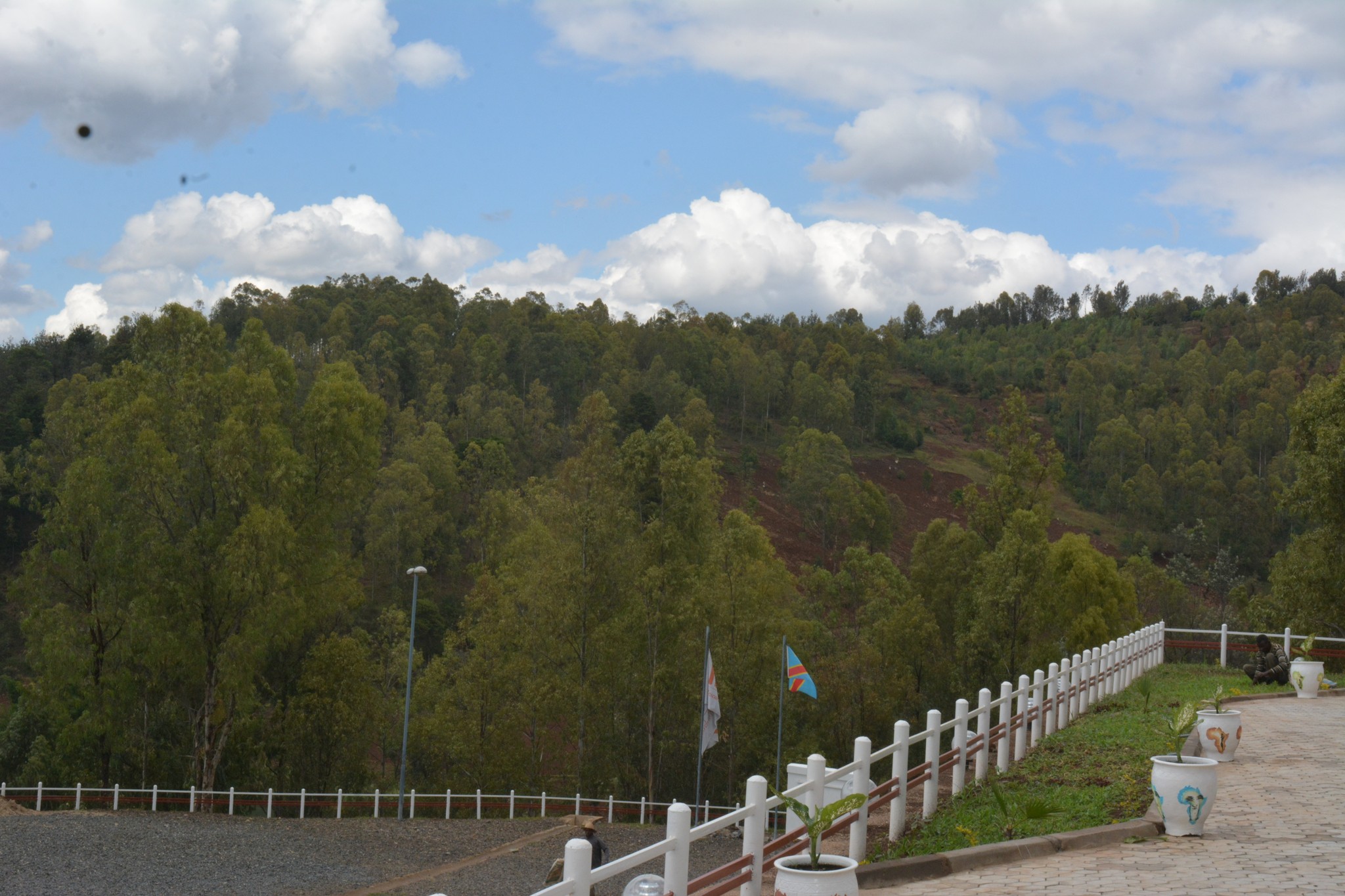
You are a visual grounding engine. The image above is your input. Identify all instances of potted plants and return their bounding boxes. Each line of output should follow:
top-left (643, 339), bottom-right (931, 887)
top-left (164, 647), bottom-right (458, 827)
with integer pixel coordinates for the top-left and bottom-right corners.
top-left (1195, 683), bottom-right (1243, 762)
top-left (766, 782), bottom-right (867, 896)
top-left (1288, 630), bottom-right (1326, 700)
top-left (1147, 702), bottom-right (1222, 838)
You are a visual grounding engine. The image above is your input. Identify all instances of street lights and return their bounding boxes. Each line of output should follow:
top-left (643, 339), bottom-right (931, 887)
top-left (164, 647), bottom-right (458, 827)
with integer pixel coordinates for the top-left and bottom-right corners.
top-left (397, 566), bottom-right (430, 825)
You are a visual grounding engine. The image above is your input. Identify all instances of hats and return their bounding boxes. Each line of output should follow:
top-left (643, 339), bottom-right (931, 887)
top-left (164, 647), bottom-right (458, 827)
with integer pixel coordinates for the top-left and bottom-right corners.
top-left (581, 822), bottom-right (597, 831)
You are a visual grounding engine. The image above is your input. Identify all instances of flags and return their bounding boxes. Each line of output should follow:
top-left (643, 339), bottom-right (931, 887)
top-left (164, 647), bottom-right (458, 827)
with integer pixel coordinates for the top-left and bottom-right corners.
top-left (786, 644), bottom-right (818, 699)
top-left (703, 648), bottom-right (720, 757)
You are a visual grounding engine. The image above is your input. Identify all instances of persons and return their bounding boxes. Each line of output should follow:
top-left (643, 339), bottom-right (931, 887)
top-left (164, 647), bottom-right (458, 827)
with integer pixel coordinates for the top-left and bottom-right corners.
top-left (1243, 634), bottom-right (1291, 684)
top-left (581, 822), bottom-right (609, 896)
